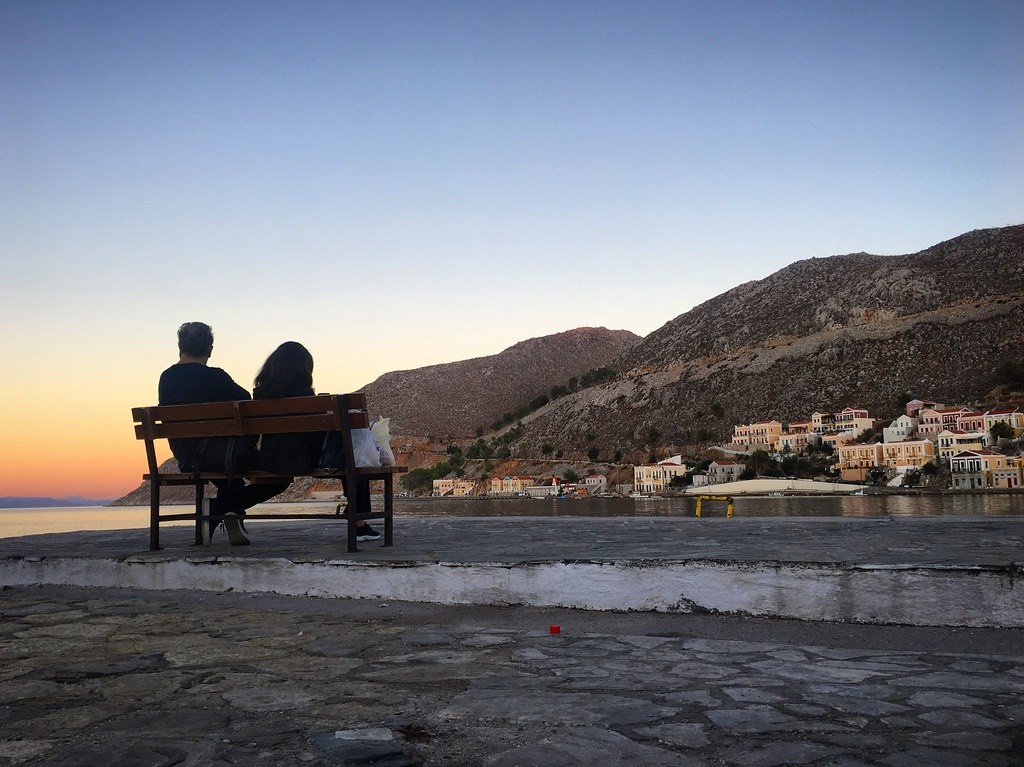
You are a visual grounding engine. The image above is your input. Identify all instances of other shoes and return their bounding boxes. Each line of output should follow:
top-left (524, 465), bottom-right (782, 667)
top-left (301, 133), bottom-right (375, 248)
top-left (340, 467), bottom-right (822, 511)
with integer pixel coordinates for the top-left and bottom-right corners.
top-left (343, 504), bottom-right (348, 515)
top-left (224, 505), bottom-right (253, 546)
top-left (356, 522), bottom-right (382, 541)
top-left (202, 497), bottom-right (224, 546)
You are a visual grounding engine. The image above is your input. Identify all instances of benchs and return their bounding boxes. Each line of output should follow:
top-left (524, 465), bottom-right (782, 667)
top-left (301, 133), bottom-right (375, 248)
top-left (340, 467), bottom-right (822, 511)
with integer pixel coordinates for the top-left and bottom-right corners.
top-left (131, 392), bottom-right (408, 551)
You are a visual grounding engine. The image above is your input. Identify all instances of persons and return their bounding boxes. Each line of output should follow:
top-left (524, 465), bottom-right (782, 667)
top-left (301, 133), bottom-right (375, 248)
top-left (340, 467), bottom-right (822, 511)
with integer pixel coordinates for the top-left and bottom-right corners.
top-left (252, 342), bottom-right (383, 542)
top-left (159, 322), bottom-right (294, 547)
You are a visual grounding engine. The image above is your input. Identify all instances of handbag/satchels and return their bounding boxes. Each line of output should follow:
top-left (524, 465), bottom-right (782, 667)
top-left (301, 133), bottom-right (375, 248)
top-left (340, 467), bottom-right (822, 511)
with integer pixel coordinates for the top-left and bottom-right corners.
top-left (350, 429), bottom-right (383, 468)
top-left (322, 430), bottom-right (346, 474)
top-left (369, 415), bottom-right (396, 467)
